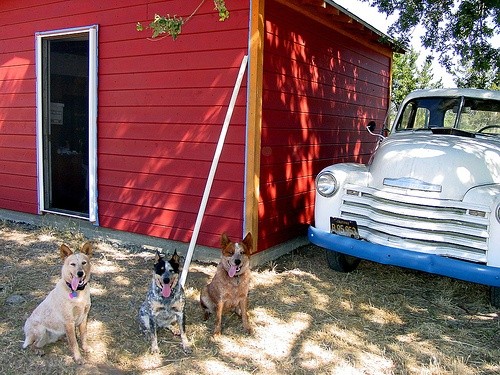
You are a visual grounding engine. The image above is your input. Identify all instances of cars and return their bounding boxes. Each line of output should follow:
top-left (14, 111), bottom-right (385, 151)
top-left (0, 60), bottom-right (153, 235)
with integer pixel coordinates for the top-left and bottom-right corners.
top-left (306, 85), bottom-right (500, 307)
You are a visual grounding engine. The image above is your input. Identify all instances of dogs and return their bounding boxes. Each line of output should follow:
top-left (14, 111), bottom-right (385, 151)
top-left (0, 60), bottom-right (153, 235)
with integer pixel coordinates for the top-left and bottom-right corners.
top-left (138, 249), bottom-right (192, 354)
top-left (200, 232), bottom-right (254, 337)
top-left (22, 241), bottom-right (92, 365)
